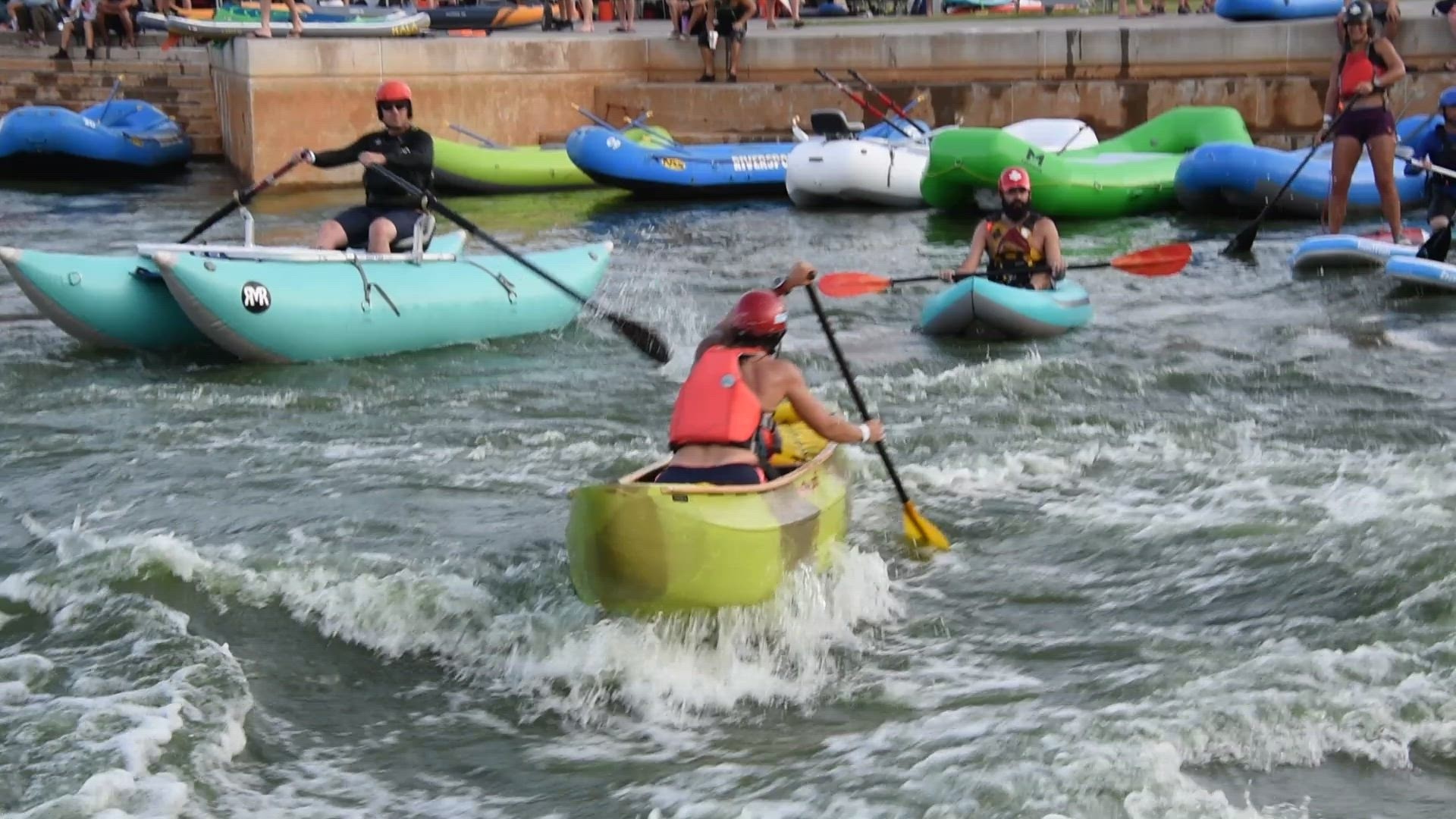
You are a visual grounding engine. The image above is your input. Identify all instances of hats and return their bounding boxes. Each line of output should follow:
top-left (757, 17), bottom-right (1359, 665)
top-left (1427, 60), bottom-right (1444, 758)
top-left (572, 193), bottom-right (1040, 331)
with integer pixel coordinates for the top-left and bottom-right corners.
top-left (999, 167), bottom-right (1032, 194)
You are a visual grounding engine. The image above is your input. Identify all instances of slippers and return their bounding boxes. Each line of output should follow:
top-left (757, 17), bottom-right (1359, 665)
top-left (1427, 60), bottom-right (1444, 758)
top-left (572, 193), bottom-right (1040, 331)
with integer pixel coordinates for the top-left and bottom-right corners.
top-left (286, 31), bottom-right (304, 38)
top-left (245, 31), bottom-right (271, 38)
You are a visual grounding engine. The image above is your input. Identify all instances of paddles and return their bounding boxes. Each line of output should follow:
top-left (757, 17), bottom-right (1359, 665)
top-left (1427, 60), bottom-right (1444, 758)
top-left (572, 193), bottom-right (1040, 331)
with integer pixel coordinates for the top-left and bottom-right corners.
top-left (370, 159), bottom-right (668, 364)
top-left (176, 150), bottom-right (308, 243)
top-left (817, 242), bottom-right (1191, 298)
top-left (618, 110), bottom-right (653, 133)
top-left (847, 68), bottom-right (925, 133)
top-left (107, 105), bottom-right (177, 132)
top-left (98, 74), bottom-right (123, 123)
top-left (642, 135), bottom-right (689, 154)
top-left (814, 67), bottom-right (922, 144)
top-left (804, 270), bottom-right (950, 550)
top-left (1395, 154), bottom-right (1456, 178)
top-left (623, 116), bottom-right (681, 147)
top-left (1223, 92), bottom-right (1361, 254)
top-left (570, 102), bottom-right (620, 133)
top-left (441, 119), bottom-right (495, 147)
top-left (858, 95), bottom-right (931, 140)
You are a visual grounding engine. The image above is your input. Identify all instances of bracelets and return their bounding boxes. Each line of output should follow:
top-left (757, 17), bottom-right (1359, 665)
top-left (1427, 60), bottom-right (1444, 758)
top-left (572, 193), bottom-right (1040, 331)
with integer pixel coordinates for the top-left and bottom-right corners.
top-left (860, 423), bottom-right (870, 443)
top-left (1323, 114), bottom-right (1333, 122)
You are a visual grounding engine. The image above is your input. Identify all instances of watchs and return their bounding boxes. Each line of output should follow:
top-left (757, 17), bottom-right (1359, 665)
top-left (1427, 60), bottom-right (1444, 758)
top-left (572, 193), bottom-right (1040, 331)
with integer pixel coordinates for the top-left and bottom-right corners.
top-left (1372, 78), bottom-right (1381, 87)
top-left (770, 275), bottom-right (792, 296)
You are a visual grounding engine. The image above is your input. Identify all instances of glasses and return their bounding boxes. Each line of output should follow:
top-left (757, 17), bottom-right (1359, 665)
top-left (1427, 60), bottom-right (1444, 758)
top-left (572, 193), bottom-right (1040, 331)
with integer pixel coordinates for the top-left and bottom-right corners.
top-left (380, 100), bottom-right (408, 110)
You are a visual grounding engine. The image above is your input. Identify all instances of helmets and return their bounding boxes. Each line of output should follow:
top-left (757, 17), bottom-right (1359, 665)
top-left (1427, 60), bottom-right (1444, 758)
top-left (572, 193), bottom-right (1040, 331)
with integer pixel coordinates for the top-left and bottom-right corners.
top-left (375, 80), bottom-right (413, 120)
top-left (1344, 0), bottom-right (1375, 24)
top-left (732, 291), bottom-right (791, 334)
top-left (1439, 88), bottom-right (1456, 108)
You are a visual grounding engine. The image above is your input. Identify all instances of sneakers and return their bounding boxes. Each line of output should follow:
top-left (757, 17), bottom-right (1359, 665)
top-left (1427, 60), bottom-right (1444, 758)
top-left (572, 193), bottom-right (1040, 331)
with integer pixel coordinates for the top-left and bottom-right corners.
top-left (85, 49), bottom-right (95, 59)
top-left (49, 46), bottom-right (69, 59)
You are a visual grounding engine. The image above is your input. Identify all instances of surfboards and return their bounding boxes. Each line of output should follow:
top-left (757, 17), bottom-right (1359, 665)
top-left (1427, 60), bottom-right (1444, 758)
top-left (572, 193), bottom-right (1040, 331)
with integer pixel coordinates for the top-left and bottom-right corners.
top-left (135, 9), bottom-right (408, 30)
top-left (1290, 228), bottom-right (1429, 268)
top-left (1385, 255), bottom-right (1456, 289)
top-left (162, 11), bottom-right (430, 37)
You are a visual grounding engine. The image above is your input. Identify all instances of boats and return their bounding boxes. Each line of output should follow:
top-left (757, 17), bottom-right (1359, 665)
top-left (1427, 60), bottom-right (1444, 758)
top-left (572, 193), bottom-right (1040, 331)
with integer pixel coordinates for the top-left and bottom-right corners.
top-left (564, 114), bottom-right (934, 194)
top-left (0, 75), bottom-right (192, 182)
top-left (136, 1), bottom-right (580, 43)
top-left (426, 121), bottom-right (677, 196)
top-left (782, 108), bottom-right (1100, 209)
top-left (0, 183), bottom-right (616, 366)
top-left (918, 104), bottom-right (1258, 221)
top-left (920, 267), bottom-right (1091, 339)
top-left (1173, 109), bottom-right (1445, 226)
top-left (562, 397), bottom-right (852, 621)
top-left (1211, 0), bottom-right (1345, 21)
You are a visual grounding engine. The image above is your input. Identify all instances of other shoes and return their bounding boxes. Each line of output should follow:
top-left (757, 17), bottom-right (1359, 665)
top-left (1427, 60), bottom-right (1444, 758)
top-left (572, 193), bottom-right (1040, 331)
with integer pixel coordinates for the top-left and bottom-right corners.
top-left (794, 20), bottom-right (805, 28)
top-left (697, 73), bottom-right (716, 82)
top-left (728, 74), bottom-right (737, 82)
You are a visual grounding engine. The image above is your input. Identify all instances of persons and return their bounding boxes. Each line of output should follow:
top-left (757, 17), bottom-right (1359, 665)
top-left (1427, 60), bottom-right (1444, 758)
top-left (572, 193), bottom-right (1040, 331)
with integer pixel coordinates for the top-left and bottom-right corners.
top-left (939, 166), bottom-right (1068, 291)
top-left (1117, 0), bottom-right (1216, 20)
top-left (0, 0), bottom-right (806, 84)
top-left (290, 81), bottom-right (436, 254)
top-left (1312, 0), bottom-right (1406, 245)
top-left (1333, 0), bottom-right (1401, 55)
top-left (1404, 83), bottom-right (1456, 263)
top-left (1432, 0), bottom-right (1456, 38)
top-left (655, 261), bottom-right (886, 486)
